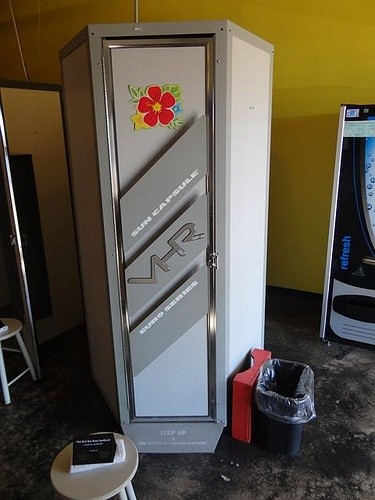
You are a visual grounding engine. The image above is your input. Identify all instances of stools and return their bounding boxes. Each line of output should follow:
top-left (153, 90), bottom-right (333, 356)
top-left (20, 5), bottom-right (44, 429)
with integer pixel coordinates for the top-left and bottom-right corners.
top-left (0, 317), bottom-right (36, 405)
top-left (51, 431), bottom-right (140, 500)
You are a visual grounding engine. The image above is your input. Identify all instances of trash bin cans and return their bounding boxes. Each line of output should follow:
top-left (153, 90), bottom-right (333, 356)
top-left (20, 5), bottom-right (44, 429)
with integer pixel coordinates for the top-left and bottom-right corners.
top-left (256, 359), bottom-right (314, 455)
